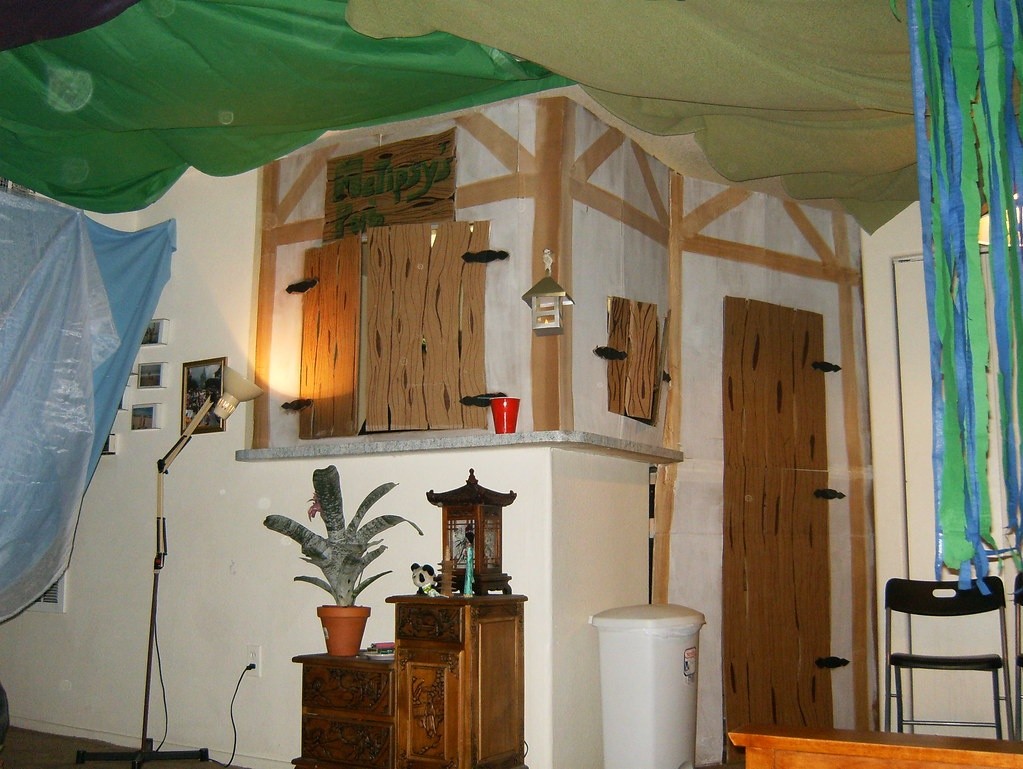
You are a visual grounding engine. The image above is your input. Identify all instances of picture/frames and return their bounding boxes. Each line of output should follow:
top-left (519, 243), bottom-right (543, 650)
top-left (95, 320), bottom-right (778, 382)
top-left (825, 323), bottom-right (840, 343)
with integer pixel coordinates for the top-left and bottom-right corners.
top-left (180, 356), bottom-right (228, 436)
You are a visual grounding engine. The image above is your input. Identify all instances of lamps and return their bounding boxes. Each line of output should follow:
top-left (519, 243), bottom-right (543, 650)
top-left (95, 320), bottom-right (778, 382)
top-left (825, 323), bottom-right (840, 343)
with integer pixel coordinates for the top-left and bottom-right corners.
top-left (76, 364), bottom-right (265, 768)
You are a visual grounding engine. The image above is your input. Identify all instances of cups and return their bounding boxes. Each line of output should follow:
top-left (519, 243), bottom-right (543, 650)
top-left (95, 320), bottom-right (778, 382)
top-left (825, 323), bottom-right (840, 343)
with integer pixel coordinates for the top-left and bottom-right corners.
top-left (489, 397), bottom-right (521, 434)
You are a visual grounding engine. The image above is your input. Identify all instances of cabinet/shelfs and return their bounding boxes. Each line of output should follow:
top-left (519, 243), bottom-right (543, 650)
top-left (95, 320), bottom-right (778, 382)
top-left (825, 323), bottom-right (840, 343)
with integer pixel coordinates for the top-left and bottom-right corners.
top-left (386, 595), bottom-right (531, 769)
top-left (291, 656), bottom-right (398, 769)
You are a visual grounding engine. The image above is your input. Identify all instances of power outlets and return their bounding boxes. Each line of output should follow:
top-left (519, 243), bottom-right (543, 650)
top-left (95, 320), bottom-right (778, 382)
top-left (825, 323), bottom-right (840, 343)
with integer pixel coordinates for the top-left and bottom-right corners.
top-left (245, 645), bottom-right (263, 676)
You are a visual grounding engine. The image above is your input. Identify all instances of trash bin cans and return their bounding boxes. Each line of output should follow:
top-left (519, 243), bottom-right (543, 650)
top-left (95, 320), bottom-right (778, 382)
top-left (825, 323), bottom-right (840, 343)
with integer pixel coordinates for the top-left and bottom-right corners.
top-left (591, 603), bottom-right (707, 769)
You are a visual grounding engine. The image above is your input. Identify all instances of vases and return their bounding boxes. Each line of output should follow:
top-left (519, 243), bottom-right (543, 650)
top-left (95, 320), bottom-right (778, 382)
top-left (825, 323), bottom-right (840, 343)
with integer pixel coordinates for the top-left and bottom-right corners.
top-left (316, 605), bottom-right (372, 657)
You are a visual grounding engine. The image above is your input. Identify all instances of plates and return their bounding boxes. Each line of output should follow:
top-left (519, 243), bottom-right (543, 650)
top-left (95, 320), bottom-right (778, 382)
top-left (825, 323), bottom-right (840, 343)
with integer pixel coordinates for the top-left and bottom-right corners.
top-left (365, 653), bottom-right (394, 660)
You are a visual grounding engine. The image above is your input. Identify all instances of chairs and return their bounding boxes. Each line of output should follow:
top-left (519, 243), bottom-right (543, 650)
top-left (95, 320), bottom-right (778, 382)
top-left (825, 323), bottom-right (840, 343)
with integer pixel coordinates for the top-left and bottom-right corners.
top-left (1015, 570), bottom-right (1023, 742)
top-left (885, 576), bottom-right (1015, 740)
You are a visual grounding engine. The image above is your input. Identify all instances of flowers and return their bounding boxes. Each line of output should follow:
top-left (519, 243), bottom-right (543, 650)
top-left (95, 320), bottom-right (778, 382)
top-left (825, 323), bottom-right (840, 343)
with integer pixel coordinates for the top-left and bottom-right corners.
top-left (262, 464), bottom-right (424, 607)
